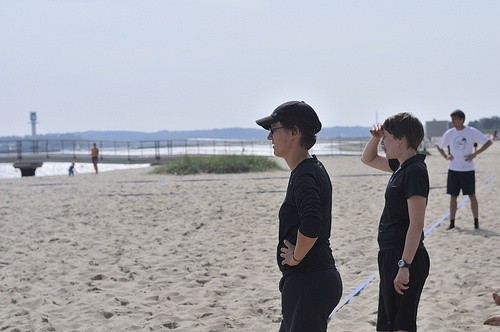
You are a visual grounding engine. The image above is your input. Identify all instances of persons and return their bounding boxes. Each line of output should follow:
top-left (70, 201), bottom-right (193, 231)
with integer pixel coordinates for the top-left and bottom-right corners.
top-left (91, 142), bottom-right (99, 174)
top-left (68, 162), bottom-right (75, 176)
top-left (255, 100), bottom-right (342, 332)
top-left (434, 109), bottom-right (493, 231)
top-left (360, 112), bottom-right (430, 332)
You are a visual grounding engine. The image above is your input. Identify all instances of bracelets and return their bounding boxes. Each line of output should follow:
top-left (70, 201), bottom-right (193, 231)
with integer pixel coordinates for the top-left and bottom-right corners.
top-left (292, 250), bottom-right (302, 262)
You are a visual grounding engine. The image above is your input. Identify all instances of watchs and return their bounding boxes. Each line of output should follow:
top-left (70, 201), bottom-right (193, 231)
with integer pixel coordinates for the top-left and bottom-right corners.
top-left (397, 259), bottom-right (411, 268)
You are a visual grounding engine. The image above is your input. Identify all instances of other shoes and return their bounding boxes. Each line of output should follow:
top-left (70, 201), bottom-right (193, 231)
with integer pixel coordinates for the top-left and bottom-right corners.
top-left (444, 224), bottom-right (455, 232)
top-left (474, 223), bottom-right (479, 231)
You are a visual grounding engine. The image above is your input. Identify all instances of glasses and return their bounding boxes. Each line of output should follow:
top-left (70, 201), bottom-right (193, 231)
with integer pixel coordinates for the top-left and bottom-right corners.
top-left (271, 126), bottom-right (294, 135)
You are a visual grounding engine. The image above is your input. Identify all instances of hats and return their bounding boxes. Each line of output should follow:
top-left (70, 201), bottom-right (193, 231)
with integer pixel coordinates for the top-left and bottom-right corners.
top-left (255, 101), bottom-right (321, 136)
